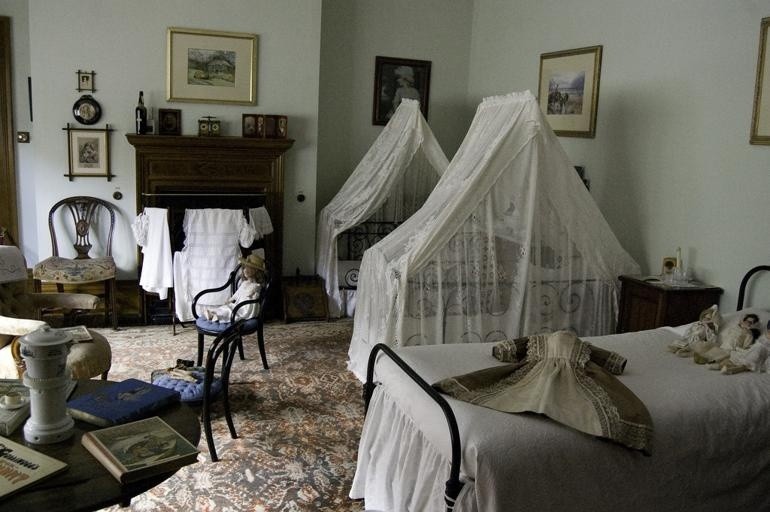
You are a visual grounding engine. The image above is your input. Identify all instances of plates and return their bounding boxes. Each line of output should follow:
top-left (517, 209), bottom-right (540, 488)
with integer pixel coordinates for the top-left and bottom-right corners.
top-left (0, 394), bottom-right (29, 410)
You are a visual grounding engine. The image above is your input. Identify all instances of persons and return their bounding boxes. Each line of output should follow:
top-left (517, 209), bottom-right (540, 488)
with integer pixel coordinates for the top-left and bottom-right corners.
top-left (692, 311), bottom-right (760, 365)
top-left (382, 63), bottom-right (423, 122)
top-left (82, 143), bottom-right (98, 163)
top-left (202, 255), bottom-right (267, 324)
top-left (670, 302), bottom-right (721, 358)
top-left (710, 320), bottom-right (770, 373)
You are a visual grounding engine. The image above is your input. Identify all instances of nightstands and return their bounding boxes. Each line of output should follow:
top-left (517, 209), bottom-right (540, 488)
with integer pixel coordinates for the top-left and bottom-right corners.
top-left (615, 271), bottom-right (724, 332)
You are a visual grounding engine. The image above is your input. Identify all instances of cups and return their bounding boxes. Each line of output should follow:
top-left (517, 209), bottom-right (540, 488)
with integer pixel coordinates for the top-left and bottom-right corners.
top-left (674, 268), bottom-right (689, 284)
top-left (5, 391), bottom-right (22, 405)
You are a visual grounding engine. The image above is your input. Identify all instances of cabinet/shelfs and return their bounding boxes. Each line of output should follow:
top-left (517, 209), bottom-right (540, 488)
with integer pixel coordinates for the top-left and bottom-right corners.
top-left (124, 131), bottom-right (297, 326)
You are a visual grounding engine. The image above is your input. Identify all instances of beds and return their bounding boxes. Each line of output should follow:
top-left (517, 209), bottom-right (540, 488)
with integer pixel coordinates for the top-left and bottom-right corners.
top-left (363, 264), bottom-right (770, 511)
top-left (360, 256), bottom-right (624, 364)
top-left (322, 218), bottom-right (485, 319)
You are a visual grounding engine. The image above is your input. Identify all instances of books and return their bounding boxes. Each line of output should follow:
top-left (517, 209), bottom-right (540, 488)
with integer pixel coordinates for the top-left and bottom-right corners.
top-left (0, 435), bottom-right (71, 496)
top-left (58, 324), bottom-right (93, 344)
top-left (81, 412), bottom-right (202, 486)
top-left (65, 377), bottom-right (180, 424)
top-left (0, 396), bottom-right (31, 437)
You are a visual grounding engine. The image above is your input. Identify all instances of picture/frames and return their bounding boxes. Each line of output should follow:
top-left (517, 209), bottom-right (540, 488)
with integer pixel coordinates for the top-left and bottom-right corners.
top-left (72, 94), bottom-right (102, 125)
top-left (75, 69), bottom-right (96, 93)
top-left (749, 17), bottom-right (770, 148)
top-left (62, 123), bottom-right (116, 181)
top-left (166, 27), bottom-right (258, 107)
top-left (538, 46), bottom-right (602, 138)
top-left (372, 56), bottom-right (432, 126)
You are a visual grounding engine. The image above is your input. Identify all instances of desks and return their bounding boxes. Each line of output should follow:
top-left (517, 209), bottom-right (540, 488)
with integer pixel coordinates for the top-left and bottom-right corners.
top-left (0, 379), bottom-right (201, 510)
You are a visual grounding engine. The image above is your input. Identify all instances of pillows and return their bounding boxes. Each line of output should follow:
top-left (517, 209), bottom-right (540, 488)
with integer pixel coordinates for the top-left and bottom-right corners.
top-left (195, 316), bottom-right (258, 333)
top-left (152, 369), bottom-right (222, 402)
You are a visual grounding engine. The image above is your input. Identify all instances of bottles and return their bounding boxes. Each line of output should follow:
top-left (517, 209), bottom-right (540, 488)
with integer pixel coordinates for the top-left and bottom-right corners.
top-left (135, 91), bottom-right (148, 136)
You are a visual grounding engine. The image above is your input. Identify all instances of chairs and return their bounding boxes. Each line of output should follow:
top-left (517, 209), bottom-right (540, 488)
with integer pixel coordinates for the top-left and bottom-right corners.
top-left (192, 264), bottom-right (274, 371)
top-left (150, 325), bottom-right (241, 462)
top-left (33, 196), bottom-right (119, 330)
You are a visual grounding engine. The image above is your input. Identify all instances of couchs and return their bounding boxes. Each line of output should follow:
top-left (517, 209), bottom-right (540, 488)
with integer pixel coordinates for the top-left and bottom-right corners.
top-left (0, 245), bottom-right (111, 382)
